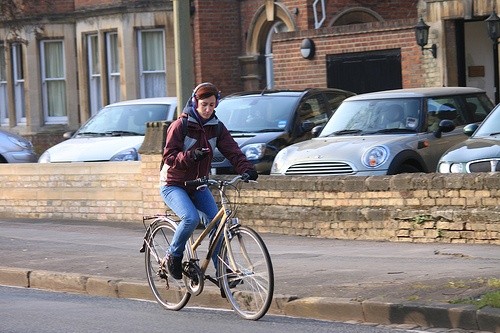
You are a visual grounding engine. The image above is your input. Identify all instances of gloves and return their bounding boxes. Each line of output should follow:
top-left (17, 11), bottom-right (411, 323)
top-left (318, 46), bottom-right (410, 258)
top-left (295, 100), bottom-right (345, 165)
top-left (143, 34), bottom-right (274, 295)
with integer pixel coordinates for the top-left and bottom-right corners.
top-left (242, 169), bottom-right (258, 183)
top-left (193, 148), bottom-right (210, 160)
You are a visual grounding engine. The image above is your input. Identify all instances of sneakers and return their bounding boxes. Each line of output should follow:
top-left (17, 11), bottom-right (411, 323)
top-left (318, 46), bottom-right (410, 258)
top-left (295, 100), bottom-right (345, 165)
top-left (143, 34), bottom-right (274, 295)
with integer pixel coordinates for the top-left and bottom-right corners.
top-left (221, 270), bottom-right (245, 298)
top-left (166, 252), bottom-right (183, 280)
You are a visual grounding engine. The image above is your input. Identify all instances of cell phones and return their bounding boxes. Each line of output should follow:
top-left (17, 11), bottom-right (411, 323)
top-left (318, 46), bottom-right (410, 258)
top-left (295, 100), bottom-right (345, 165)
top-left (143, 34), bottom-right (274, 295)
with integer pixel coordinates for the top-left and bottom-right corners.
top-left (201, 148), bottom-right (209, 151)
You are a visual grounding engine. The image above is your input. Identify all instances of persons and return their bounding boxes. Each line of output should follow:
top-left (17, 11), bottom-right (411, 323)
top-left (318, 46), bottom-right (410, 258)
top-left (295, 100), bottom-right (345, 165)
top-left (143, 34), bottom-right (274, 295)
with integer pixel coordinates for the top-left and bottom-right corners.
top-left (160, 82), bottom-right (258, 299)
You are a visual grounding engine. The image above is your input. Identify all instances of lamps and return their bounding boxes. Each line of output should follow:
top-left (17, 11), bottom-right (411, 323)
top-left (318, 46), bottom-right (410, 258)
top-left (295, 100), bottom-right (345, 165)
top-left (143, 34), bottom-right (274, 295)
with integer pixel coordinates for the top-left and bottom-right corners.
top-left (414, 18), bottom-right (436, 58)
top-left (485, 10), bottom-right (500, 44)
top-left (300, 38), bottom-right (315, 59)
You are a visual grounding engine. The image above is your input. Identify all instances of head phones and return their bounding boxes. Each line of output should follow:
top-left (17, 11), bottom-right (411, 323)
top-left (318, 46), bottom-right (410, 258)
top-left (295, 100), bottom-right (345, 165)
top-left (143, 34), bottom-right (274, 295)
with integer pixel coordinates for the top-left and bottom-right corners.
top-left (191, 82), bottom-right (220, 108)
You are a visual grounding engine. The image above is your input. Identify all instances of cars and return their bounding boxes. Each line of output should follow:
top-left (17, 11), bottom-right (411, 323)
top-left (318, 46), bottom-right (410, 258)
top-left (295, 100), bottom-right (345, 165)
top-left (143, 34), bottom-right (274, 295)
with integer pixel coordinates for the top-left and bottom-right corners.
top-left (38, 97), bottom-right (178, 163)
top-left (0, 128), bottom-right (39, 164)
top-left (208, 87), bottom-right (358, 176)
top-left (269, 87), bottom-right (497, 176)
top-left (435, 102), bottom-right (500, 173)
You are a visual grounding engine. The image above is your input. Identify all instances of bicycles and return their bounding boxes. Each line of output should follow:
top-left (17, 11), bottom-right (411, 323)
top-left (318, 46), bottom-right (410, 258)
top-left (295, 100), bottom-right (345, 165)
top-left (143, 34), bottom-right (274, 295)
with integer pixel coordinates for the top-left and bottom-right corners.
top-left (139, 172), bottom-right (275, 321)
